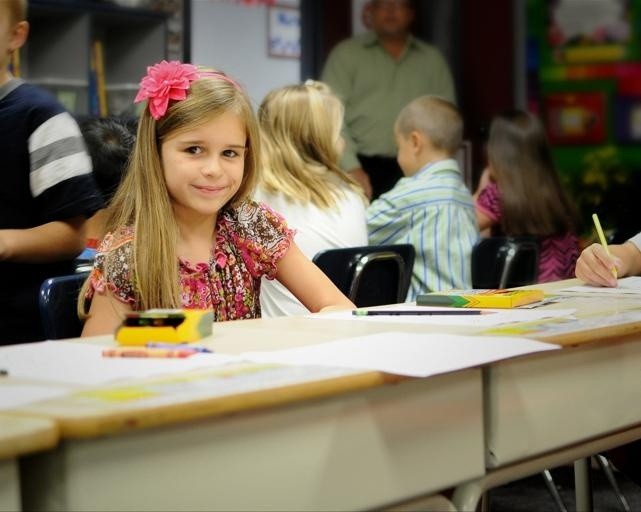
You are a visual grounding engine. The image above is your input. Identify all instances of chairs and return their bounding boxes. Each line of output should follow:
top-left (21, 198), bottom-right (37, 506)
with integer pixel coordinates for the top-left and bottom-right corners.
top-left (472, 234), bottom-right (634, 512)
top-left (36, 271), bottom-right (94, 337)
top-left (312, 244), bottom-right (415, 307)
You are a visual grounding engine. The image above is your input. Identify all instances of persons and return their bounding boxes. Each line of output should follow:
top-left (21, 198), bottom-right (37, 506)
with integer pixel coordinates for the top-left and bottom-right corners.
top-left (75, 61), bottom-right (359, 337)
top-left (0, 0), bottom-right (93, 348)
top-left (78, 110), bottom-right (139, 208)
top-left (246, 79), bottom-right (372, 317)
top-left (575, 231), bottom-right (641, 288)
top-left (471, 109), bottom-right (585, 282)
top-left (318, 1), bottom-right (458, 201)
top-left (365, 95), bottom-right (482, 303)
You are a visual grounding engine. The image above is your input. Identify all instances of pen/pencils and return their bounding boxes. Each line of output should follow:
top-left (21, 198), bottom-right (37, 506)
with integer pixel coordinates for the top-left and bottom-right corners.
top-left (137, 319), bottom-right (166, 326)
top-left (102, 350), bottom-right (194, 358)
top-left (352, 310), bottom-right (498, 315)
top-left (592, 213), bottom-right (618, 279)
top-left (147, 342), bottom-right (213, 352)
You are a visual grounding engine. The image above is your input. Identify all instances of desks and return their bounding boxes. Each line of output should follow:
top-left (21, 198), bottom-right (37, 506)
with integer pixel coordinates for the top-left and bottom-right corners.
top-left (0, 314), bottom-right (485, 512)
top-left (380, 270), bottom-right (640, 512)
top-left (1, 410), bottom-right (60, 512)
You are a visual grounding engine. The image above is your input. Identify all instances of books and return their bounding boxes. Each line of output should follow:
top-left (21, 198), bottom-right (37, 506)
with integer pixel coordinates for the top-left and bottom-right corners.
top-left (415, 288), bottom-right (544, 308)
top-left (113, 307), bottom-right (214, 346)
